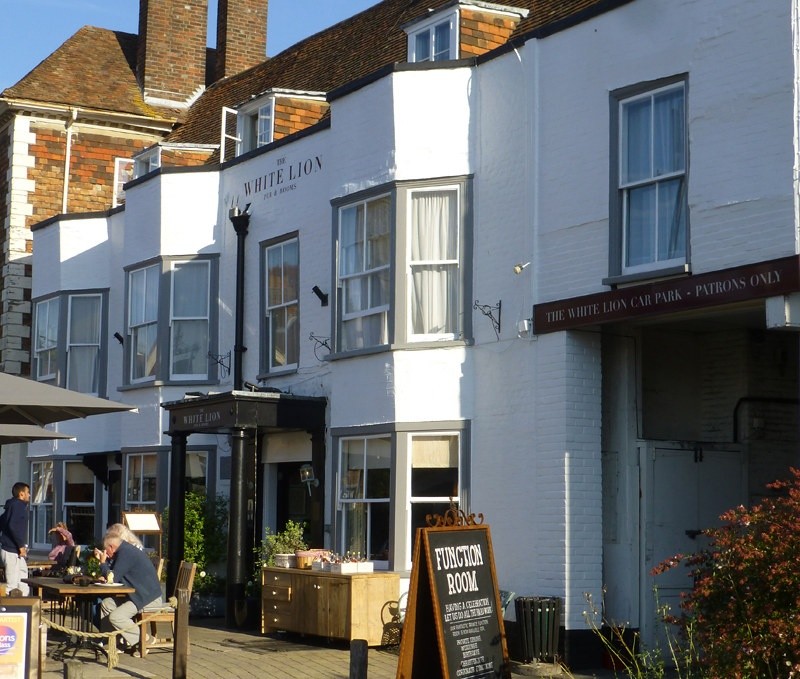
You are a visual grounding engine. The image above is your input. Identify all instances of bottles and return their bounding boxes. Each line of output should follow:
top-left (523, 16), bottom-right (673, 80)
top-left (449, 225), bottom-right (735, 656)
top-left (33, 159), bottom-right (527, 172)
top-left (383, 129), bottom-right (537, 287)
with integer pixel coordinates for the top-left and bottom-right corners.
top-left (346, 550), bottom-right (350, 562)
top-left (356, 552), bottom-right (361, 561)
top-left (352, 552), bottom-right (356, 562)
top-left (20, 544), bottom-right (30, 557)
top-left (335, 553), bottom-right (340, 563)
top-left (330, 550), bottom-right (334, 560)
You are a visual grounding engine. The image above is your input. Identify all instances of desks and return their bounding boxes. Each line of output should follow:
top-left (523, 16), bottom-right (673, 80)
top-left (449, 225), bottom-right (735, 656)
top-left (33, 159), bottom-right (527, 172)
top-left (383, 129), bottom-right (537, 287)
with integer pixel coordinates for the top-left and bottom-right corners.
top-left (20, 578), bottom-right (135, 661)
top-left (25, 559), bottom-right (58, 609)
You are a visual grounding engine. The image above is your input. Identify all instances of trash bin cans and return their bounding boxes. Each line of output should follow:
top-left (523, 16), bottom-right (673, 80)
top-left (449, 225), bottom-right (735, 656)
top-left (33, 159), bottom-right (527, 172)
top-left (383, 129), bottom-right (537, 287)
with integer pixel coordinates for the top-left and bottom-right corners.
top-left (515, 596), bottom-right (558, 661)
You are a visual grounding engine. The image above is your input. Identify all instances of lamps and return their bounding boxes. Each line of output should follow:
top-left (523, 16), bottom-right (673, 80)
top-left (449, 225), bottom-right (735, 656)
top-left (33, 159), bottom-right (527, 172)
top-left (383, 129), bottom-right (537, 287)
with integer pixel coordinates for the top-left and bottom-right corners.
top-left (299, 464), bottom-right (319, 496)
top-left (513, 261), bottom-right (531, 274)
top-left (229, 207), bottom-right (242, 219)
top-left (517, 319), bottom-right (531, 333)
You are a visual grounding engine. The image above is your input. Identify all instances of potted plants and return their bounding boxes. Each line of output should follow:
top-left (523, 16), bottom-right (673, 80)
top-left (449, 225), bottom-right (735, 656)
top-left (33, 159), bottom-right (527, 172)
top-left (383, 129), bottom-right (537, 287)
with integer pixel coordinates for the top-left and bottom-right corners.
top-left (160, 486), bottom-right (229, 618)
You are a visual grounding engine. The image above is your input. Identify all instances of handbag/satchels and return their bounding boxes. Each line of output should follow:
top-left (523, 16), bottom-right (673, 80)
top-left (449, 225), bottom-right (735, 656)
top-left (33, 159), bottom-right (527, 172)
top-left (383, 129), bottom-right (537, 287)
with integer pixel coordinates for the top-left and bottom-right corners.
top-left (63, 574), bottom-right (88, 585)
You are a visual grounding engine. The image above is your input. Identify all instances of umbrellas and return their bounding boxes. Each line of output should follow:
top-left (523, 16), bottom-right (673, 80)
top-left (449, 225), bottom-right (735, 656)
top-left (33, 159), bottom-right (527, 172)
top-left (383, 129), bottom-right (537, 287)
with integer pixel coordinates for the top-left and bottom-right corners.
top-left (0, 370), bottom-right (135, 425)
top-left (0, 424), bottom-right (75, 443)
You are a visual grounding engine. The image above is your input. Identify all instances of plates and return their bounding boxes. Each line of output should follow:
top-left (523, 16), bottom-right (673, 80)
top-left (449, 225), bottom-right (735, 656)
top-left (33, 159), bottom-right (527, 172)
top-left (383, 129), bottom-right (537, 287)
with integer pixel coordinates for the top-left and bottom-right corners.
top-left (94, 583), bottom-right (124, 587)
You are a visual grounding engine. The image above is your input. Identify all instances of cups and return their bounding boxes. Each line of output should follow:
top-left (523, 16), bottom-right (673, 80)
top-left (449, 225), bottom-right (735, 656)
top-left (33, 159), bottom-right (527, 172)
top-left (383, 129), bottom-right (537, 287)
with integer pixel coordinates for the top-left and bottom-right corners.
top-left (107, 570), bottom-right (115, 584)
top-left (79, 579), bottom-right (90, 587)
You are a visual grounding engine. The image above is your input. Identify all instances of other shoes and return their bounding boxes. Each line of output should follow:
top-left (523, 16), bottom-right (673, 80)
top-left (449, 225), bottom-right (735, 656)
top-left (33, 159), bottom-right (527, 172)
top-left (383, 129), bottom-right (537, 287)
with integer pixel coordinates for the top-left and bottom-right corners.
top-left (98, 643), bottom-right (126, 653)
top-left (133, 636), bottom-right (156, 658)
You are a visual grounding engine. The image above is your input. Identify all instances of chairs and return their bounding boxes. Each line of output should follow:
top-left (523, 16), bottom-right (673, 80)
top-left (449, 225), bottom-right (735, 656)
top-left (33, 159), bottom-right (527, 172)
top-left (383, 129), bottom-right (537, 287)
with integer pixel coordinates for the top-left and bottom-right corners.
top-left (133, 560), bottom-right (198, 658)
top-left (151, 558), bottom-right (165, 581)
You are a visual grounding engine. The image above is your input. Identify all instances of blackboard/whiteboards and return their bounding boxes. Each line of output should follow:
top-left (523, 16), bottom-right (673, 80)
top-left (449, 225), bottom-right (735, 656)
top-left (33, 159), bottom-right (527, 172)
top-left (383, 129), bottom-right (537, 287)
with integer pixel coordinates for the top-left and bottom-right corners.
top-left (396, 525), bottom-right (511, 679)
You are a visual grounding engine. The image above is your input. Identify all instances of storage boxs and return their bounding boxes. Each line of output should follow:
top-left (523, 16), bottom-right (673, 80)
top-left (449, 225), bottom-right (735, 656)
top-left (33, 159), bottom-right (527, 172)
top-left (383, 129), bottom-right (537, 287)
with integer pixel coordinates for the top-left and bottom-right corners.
top-left (357, 562), bottom-right (374, 574)
top-left (331, 563), bottom-right (358, 573)
top-left (323, 562), bottom-right (333, 572)
top-left (311, 562), bottom-right (323, 571)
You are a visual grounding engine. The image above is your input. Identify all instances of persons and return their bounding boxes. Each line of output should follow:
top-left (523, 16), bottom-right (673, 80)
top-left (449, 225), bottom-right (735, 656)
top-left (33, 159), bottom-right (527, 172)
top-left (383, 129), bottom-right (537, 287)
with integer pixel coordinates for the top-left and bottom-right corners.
top-left (33, 522), bottom-right (75, 577)
top-left (0, 482), bottom-right (30, 596)
top-left (85, 523), bottom-right (162, 657)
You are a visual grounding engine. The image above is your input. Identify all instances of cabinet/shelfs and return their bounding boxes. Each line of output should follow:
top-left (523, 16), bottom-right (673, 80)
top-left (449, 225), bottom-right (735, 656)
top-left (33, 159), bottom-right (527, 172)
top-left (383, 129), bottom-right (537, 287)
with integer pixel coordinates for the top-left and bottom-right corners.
top-left (260, 566), bottom-right (400, 646)
